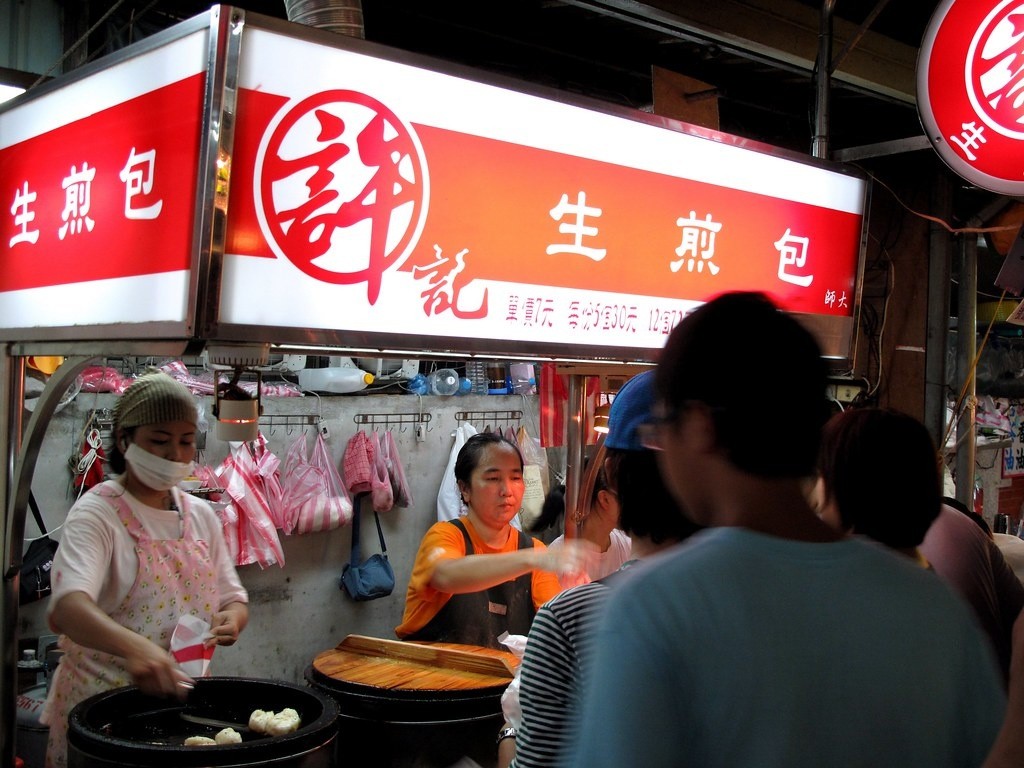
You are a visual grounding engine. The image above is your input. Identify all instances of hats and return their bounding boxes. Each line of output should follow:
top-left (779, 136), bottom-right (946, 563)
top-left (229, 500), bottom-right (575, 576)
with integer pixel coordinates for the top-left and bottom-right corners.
top-left (600, 367), bottom-right (665, 453)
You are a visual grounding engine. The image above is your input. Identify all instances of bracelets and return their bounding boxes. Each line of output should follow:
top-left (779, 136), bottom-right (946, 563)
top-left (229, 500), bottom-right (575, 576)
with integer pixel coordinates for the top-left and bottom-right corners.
top-left (496, 727), bottom-right (517, 744)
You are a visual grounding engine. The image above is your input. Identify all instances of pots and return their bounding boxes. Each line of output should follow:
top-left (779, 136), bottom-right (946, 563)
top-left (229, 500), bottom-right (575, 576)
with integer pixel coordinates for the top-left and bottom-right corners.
top-left (305, 643), bottom-right (504, 722)
top-left (68, 677), bottom-right (340, 767)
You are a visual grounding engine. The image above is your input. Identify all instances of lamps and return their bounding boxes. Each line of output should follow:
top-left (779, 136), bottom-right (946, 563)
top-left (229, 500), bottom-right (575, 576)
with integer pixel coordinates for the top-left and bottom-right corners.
top-left (593, 407), bottom-right (609, 433)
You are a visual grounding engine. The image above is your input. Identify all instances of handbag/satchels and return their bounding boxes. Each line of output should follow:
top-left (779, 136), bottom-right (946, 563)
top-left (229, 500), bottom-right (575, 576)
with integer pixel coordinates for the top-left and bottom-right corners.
top-left (517, 464), bottom-right (546, 533)
top-left (338, 553), bottom-right (396, 603)
top-left (18, 536), bottom-right (61, 606)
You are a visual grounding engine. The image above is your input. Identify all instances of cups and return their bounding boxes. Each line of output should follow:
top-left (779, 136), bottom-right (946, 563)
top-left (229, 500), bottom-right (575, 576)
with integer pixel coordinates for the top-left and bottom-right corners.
top-left (994, 513), bottom-right (1008, 535)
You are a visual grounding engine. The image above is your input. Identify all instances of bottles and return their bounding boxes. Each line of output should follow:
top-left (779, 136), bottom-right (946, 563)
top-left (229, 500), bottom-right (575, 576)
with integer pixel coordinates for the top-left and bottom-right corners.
top-left (489, 375), bottom-right (536, 396)
top-left (298, 367), bottom-right (374, 393)
top-left (456, 377), bottom-right (473, 396)
top-left (428, 369), bottom-right (459, 395)
top-left (18, 649), bottom-right (45, 692)
top-left (467, 362), bottom-right (488, 396)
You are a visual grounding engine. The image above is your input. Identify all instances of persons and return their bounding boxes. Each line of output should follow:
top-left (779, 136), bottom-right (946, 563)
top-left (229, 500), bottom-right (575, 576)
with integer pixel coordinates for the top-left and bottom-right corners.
top-left (39, 365), bottom-right (249, 768)
top-left (574, 290), bottom-right (1007, 768)
top-left (496, 366), bottom-right (1024, 768)
top-left (396, 433), bottom-right (601, 654)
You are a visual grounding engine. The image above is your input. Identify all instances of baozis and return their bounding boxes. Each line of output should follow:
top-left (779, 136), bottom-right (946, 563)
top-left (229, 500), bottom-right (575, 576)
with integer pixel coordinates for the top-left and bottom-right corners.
top-left (215, 727), bottom-right (242, 745)
top-left (249, 707), bottom-right (301, 738)
top-left (184, 736), bottom-right (217, 745)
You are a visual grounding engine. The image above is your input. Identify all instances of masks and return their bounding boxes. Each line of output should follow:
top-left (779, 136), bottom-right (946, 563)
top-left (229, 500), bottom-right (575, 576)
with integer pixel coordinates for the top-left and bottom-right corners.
top-left (122, 441), bottom-right (199, 492)
